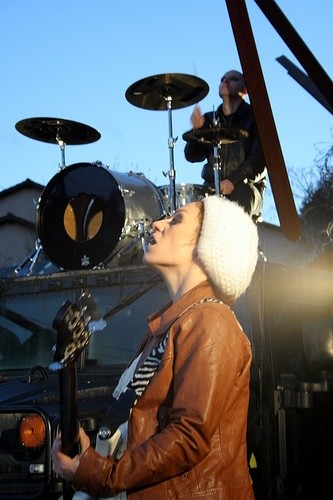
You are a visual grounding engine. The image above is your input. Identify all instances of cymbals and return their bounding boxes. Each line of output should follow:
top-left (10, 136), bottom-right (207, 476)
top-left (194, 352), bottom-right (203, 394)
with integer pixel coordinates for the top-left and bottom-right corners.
top-left (15, 117), bottom-right (101, 146)
top-left (125, 73), bottom-right (209, 111)
top-left (182, 127), bottom-right (249, 146)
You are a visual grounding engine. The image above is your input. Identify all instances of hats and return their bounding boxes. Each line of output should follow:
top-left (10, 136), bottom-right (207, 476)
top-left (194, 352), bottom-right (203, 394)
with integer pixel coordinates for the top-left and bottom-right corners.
top-left (196, 195), bottom-right (259, 304)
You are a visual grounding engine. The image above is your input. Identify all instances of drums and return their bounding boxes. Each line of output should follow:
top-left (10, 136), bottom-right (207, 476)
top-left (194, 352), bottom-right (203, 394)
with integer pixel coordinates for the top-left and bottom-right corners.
top-left (158, 183), bottom-right (215, 217)
top-left (36, 162), bottom-right (168, 270)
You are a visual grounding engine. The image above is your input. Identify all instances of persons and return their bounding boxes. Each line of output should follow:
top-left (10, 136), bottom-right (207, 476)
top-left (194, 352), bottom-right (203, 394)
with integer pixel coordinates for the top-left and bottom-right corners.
top-left (52, 195), bottom-right (259, 500)
top-left (184, 70), bottom-right (268, 221)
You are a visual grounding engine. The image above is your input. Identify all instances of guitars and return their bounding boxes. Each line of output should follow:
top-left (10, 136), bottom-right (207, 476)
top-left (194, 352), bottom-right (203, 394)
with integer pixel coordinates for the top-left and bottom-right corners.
top-left (53, 292), bottom-right (100, 500)
